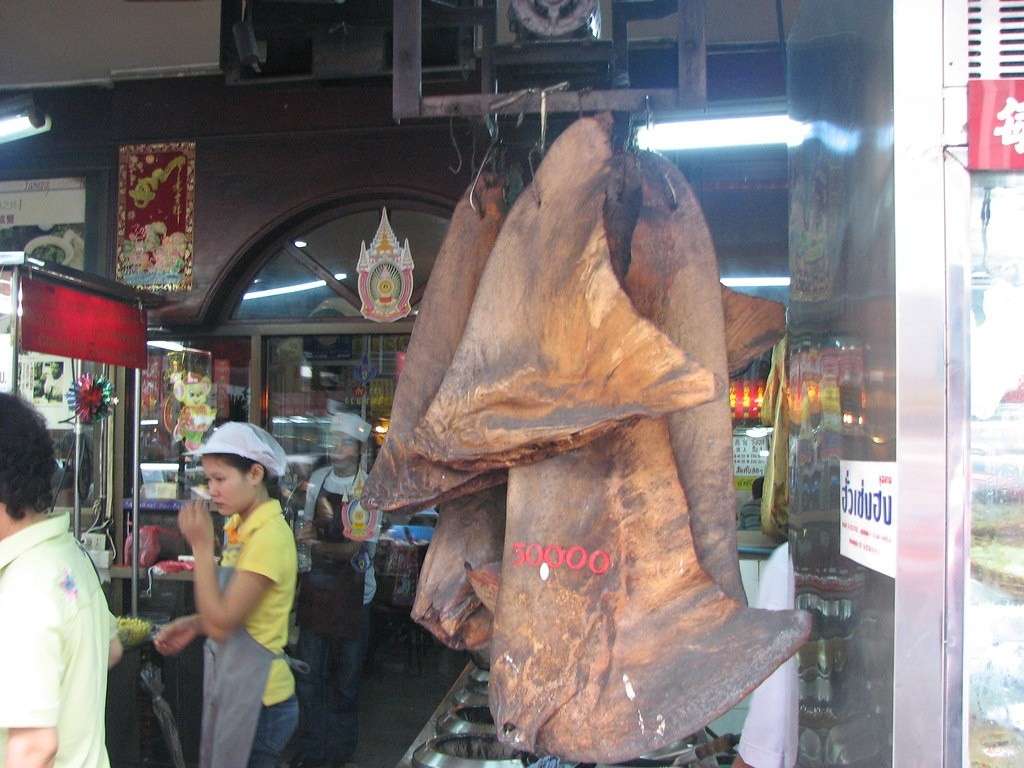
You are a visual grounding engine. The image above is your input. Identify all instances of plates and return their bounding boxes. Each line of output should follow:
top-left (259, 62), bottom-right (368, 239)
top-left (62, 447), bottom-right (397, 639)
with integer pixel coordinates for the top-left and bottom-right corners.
top-left (24, 236), bottom-right (73, 265)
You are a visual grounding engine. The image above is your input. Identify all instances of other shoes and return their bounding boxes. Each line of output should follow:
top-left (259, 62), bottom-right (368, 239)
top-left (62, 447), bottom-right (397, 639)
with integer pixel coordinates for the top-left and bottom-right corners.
top-left (328, 753), bottom-right (359, 768)
top-left (277, 752), bottom-right (325, 768)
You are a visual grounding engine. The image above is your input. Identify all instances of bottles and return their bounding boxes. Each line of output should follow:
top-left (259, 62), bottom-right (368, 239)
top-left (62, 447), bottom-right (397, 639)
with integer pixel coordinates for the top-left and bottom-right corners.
top-left (295, 510), bottom-right (311, 572)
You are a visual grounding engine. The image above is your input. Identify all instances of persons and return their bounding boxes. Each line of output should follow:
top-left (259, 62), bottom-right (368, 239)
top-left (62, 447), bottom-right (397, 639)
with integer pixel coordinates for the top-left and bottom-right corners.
top-left (155, 423), bottom-right (299, 768)
top-left (293, 412), bottom-right (382, 768)
top-left (0, 392), bottom-right (122, 768)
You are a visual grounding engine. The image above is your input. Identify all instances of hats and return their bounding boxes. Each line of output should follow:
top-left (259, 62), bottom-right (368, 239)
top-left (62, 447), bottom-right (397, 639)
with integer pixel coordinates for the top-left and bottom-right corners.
top-left (181, 421), bottom-right (287, 476)
top-left (331, 411), bottom-right (373, 442)
